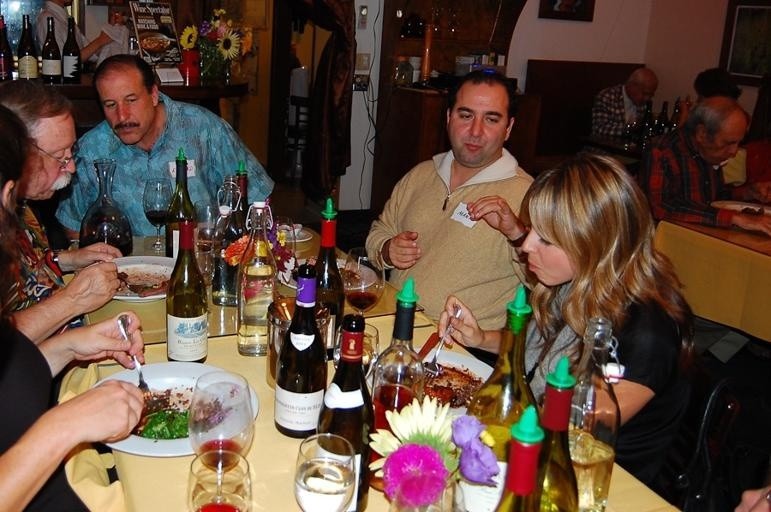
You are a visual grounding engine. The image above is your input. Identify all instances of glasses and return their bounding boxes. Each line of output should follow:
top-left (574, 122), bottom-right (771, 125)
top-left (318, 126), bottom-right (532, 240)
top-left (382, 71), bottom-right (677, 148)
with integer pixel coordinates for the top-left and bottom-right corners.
top-left (29, 140), bottom-right (79, 168)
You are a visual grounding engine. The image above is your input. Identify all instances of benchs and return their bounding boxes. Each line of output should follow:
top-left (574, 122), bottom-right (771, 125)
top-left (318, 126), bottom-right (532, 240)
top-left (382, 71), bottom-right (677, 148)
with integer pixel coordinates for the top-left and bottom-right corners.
top-left (524, 59), bottom-right (647, 179)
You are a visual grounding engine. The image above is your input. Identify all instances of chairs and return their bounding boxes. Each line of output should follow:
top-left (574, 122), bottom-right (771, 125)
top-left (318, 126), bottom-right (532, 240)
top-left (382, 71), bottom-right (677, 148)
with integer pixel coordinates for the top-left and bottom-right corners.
top-left (613, 314), bottom-right (771, 511)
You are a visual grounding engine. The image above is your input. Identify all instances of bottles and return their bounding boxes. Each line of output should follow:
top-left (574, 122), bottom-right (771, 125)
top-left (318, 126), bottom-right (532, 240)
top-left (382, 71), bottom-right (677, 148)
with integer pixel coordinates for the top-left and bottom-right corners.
top-left (62, 19), bottom-right (83, 83)
top-left (492, 357), bottom-right (581, 512)
top-left (41, 17), bottom-right (63, 84)
top-left (19, 14), bottom-right (36, 81)
top-left (318, 313), bottom-right (374, 512)
top-left (369, 278), bottom-right (427, 493)
top-left (80, 157), bottom-right (134, 257)
top-left (0, 17), bottom-right (13, 82)
top-left (570, 316), bottom-right (622, 510)
top-left (274, 264), bottom-right (328, 439)
top-left (452, 284), bottom-right (540, 511)
top-left (166, 148), bottom-right (347, 362)
top-left (623, 95), bottom-right (695, 158)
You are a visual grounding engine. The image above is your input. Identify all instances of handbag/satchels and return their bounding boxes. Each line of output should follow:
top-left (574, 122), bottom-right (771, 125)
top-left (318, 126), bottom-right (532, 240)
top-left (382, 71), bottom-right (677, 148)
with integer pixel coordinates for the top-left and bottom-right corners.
top-left (647, 377), bottom-right (769, 512)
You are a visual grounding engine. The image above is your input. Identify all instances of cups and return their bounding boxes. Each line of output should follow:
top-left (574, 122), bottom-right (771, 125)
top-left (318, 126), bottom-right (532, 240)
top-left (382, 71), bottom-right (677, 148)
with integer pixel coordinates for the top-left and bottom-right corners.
top-left (388, 476), bottom-right (449, 511)
top-left (334, 323), bottom-right (380, 380)
top-left (186, 450), bottom-right (253, 512)
top-left (294, 434), bottom-right (357, 512)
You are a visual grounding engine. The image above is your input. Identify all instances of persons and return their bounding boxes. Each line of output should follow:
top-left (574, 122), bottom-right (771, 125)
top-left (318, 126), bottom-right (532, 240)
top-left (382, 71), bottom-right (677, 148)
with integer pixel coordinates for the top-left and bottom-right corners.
top-left (591, 66), bottom-right (658, 140)
top-left (364, 71), bottom-right (535, 368)
top-left (33, 1), bottom-right (125, 71)
top-left (55, 54), bottom-right (276, 243)
top-left (438, 150), bottom-right (696, 512)
top-left (637, 95), bottom-right (771, 237)
top-left (1, 74), bottom-right (146, 512)
top-left (679, 67), bottom-right (744, 127)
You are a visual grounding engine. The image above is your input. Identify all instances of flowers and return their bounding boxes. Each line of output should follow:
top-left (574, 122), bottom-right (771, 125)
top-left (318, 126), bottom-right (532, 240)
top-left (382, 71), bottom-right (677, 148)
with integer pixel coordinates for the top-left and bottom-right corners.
top-left (179, 8), bottom-right (255, 75)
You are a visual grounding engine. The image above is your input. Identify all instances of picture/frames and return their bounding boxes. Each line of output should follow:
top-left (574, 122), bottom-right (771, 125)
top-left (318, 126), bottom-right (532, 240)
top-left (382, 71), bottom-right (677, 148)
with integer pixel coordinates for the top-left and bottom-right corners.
top-left (719, 0), bottom-right (771, 89)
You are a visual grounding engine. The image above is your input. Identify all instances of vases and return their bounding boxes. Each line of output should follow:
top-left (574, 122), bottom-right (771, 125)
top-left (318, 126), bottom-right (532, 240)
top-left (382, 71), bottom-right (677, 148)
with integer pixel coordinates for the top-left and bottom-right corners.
top-left (200, 46), bottom-right (231, 86)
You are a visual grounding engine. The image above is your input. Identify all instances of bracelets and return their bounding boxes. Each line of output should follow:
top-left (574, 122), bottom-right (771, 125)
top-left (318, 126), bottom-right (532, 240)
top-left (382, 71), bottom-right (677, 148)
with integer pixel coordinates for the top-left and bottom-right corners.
top-left (383, 239), bottom-right (392, 266)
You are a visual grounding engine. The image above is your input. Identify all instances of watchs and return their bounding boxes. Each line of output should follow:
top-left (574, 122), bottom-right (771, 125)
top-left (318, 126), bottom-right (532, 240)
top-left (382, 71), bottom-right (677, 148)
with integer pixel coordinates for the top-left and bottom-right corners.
top-left (506, 221), bottom-right (531, 247)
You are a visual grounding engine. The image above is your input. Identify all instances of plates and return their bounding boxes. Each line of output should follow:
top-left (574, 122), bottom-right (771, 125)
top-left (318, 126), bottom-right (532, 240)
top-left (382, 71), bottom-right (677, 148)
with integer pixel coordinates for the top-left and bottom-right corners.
top-left (86, 227), bottom-right (378, 304)
top-left (369, 349), bottom-right (496, 420)
top-left (92, 360), bottom-right (259, 459)
top-left (712, 200), bottom-right (771, 217)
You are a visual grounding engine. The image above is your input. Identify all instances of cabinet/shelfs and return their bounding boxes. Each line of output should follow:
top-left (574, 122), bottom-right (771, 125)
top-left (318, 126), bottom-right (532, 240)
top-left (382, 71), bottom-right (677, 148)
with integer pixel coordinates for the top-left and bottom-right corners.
top-left (370, 0), bottom-right (540, 222)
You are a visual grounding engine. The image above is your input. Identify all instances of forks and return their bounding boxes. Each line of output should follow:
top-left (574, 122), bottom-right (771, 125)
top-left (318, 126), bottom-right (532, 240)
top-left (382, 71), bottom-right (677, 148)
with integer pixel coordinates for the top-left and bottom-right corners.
top-left (115, 312), bottom-right (161, 407)
top-left (424, 304), bottom-right (462, 385)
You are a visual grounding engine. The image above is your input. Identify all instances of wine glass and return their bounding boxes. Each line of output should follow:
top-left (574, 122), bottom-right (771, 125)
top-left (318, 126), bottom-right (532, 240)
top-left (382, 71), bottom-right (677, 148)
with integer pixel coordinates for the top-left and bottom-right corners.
top-left (187, 371), bottom-right (254, 511)
top-left (343, 245), bottom-right (387, 318)
top-left (146, 178), bottom-right (173, 251)
